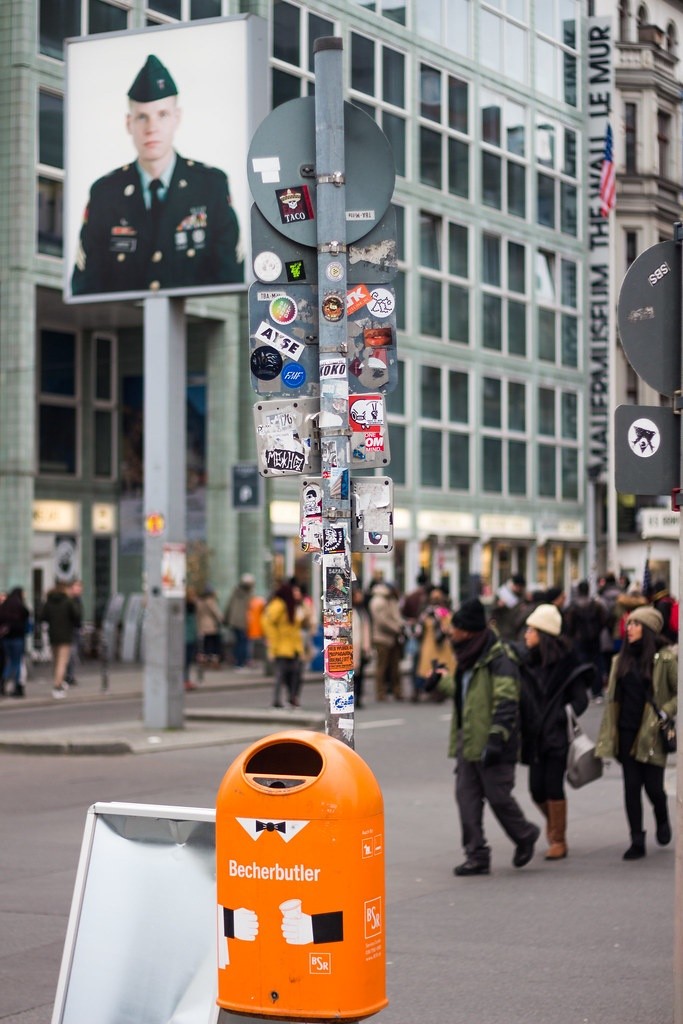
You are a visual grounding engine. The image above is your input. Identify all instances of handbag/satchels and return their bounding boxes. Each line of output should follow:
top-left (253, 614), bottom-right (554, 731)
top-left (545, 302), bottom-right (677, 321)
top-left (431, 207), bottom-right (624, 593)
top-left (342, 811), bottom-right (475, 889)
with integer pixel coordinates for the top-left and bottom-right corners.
top-left (657, 719), bottom-right (677, 754)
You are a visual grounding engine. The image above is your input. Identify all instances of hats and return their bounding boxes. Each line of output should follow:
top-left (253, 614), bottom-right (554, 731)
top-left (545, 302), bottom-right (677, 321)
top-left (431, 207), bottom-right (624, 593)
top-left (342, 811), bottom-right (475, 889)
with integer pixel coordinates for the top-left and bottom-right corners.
top-left (626, 605), bottom-right (663, 633)
top-left (126, 54), bottom-right (179, 102)
top-left (526, 603), bottom-right (562, 636)
top-left (449, 597), bottom-right (486, 632)
top-left (371, 584), bottom-right (391, 595)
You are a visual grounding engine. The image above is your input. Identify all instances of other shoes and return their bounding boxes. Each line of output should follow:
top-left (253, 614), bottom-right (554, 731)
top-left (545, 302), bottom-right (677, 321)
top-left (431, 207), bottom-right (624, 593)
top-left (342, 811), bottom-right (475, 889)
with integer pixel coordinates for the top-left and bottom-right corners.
top-left (654, 802), bottom-right (672, 845)
top-left (513, 826), bottom-right (538, 867)
top-left (454, 847), bottom-right (490, 876)
top-left (624, 831), bottom-right (647, 860)
top-left (12, 686), bottom-right (23, 696)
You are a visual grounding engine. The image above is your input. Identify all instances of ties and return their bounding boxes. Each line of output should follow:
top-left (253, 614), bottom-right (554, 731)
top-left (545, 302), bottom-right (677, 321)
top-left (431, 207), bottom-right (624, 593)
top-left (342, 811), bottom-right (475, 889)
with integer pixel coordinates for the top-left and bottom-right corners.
top-left (147, 179), bottom-right (164, 230)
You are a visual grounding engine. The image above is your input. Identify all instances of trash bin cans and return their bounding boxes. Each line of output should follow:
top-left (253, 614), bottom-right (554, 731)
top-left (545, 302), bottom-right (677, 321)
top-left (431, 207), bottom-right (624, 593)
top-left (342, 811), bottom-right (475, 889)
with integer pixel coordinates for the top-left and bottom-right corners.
top-left (215, 730), bottom-right (388, 1024)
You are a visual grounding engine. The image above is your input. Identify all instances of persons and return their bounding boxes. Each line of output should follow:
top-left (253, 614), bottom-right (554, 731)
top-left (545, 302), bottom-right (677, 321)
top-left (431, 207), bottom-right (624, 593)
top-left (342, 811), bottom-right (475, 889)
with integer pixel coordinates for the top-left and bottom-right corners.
top-left (490, 573), bottom-right (533, 643)
top-left (544, 580), bottom-right (610, 704)
top-left (450, 596), bottom-right (540, 876)
top-left (185, 584), bottom-right (223, 691)
top-left (0, 585), bottom-right (33, 699)
top-left (594, 606), bottom-right (677, 861)
top-left (416, 567), bottom-right (429, 586)
top-left (531, 589), bottom-right (544, 604)
top-left (36, 574), bottom-right (84, 697)
top-left (40, 535), bottom-right (82, 606)
top-left (223, 572), bottom-right (318, 707)
top-left (352, 570), bottom-right (457, 710)
top-left (519, 603), bottom-right (588, 860)
top-left (592, 573), bottom-right (679, 649)
top-left (71, 54), bottom-right (244, 298)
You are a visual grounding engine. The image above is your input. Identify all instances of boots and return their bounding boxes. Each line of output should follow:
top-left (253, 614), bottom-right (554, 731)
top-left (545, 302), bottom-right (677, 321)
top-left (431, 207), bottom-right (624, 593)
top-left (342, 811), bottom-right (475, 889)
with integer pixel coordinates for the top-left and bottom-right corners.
top-left (536, 800), bottom-right (567, 860)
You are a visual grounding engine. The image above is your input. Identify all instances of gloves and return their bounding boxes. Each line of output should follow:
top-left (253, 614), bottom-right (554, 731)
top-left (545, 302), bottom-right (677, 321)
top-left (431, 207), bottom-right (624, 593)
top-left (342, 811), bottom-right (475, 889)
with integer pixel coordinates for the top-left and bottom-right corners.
top-left (482, 734), bottom-right (504, 763)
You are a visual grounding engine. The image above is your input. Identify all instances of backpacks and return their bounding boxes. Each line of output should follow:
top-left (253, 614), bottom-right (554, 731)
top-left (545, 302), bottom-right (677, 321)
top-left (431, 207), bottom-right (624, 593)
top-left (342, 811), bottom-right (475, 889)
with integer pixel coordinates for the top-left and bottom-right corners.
top-left (564, 703), bottom-right (602, 788)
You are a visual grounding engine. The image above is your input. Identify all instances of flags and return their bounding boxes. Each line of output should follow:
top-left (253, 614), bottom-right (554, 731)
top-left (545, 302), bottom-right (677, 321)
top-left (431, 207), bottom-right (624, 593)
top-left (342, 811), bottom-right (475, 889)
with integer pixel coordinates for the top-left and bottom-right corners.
top-left (599, 124), bottom-right (615, 217)
